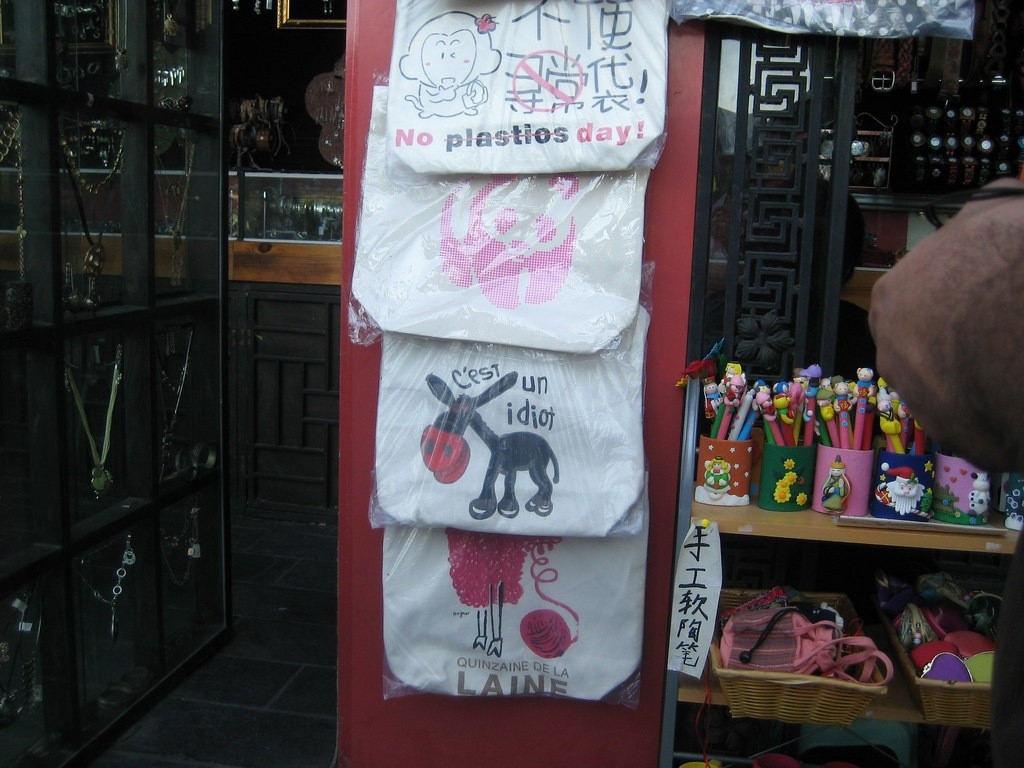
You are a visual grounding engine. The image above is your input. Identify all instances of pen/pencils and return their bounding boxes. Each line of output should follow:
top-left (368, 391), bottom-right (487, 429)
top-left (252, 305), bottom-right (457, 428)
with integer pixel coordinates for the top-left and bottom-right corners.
top-left (702, 362), bottom-right (767, 441)
top-left (816, 366), bottom-right (880, 451)
top-left (875, 375), bottom-right (930, 455)
top-left (755, 363), bottom-right (821, 446)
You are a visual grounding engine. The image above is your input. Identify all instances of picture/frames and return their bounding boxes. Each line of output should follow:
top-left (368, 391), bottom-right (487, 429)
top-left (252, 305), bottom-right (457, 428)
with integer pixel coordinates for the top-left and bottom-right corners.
top-left (275, 1), bottom-right (346, 30)
top-left (0, 0), bottom-right (114, 58)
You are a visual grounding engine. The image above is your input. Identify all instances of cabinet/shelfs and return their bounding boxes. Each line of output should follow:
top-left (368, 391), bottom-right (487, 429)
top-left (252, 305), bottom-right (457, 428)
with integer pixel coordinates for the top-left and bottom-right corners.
top-left (671, 486), bottom-right (1023, 767)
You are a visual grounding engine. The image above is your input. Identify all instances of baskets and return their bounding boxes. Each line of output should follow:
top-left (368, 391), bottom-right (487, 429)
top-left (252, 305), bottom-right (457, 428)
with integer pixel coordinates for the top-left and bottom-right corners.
top-left (874, 594), bottom-right (993, 731)
top-left (709, 586), bottom-right (888, 728)
